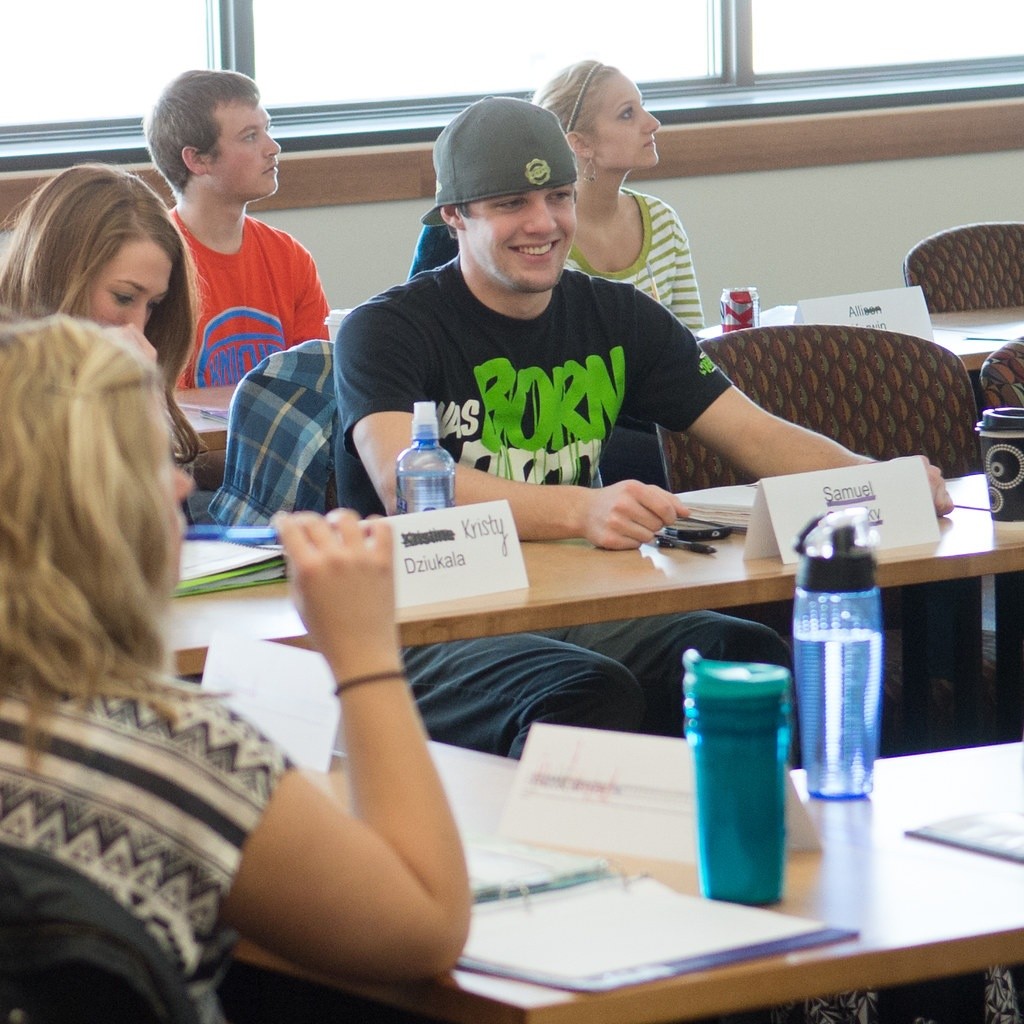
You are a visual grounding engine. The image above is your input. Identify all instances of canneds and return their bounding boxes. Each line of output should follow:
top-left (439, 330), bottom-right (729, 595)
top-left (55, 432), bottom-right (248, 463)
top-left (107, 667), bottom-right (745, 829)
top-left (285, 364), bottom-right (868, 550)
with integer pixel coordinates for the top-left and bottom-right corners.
top-left (719, 286), bottom-right (760, 333)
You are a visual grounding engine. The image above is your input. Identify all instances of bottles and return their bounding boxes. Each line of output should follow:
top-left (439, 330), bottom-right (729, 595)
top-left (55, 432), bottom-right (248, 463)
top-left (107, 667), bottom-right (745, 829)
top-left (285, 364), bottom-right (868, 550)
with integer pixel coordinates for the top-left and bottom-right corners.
top-left (396, 401), bottom-right (456, 515)
top-left (792, 506), bottom-right (884, 801)
top-left (683, 648), bottom-right (794, 906)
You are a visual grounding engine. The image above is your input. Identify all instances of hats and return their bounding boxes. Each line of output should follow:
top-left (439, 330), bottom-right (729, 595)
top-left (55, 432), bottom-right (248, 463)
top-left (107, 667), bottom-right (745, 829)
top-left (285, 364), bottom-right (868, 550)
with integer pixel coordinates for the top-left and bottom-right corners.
top-left (421, 95), bottom-right (578, 226)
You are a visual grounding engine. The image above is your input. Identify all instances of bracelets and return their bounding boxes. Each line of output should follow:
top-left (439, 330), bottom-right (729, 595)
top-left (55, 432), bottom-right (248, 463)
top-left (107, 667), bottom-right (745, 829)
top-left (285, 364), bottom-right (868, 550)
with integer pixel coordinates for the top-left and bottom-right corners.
top-left (334, 670), bottom-right (408, 695)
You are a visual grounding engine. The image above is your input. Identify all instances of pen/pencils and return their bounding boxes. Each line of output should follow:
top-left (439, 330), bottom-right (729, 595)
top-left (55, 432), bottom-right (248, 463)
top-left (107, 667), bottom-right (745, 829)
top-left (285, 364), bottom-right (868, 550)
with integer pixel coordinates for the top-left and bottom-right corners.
top-left (221, 526), bottom-right (279, 545)
top-left (655, 536), bottom-right (717, 554)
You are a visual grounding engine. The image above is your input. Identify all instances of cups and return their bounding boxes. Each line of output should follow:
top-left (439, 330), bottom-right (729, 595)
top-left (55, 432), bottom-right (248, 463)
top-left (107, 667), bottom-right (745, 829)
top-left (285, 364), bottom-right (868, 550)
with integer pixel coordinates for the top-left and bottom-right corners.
top-left (323, 309), bottom-right (355, 343)
top-left (975, 407), bottom-right (1024, 530)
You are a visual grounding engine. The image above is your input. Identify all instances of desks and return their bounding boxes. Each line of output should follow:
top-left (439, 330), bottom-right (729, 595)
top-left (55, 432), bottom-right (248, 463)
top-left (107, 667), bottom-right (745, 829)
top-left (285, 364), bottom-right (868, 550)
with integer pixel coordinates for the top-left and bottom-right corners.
top-left (692, 305), bottom-right (1024, 372)
top-left (225, 743), bottom-right (1023, 1024)
top-left (162, 471), bottom-right (1023, 751)
top-left (172, 383), bottom-right (238, 494)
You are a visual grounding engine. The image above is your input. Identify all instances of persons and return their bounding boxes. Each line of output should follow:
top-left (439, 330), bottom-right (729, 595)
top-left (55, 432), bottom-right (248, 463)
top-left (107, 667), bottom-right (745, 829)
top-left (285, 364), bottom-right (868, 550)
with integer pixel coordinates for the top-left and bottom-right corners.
top-left (0, 312), bottom-right (473, 1024)
top-left (141, 69), bottom-right (331, 390)
top-left (1, 165), bottom-right (200, 464)
top-left (332, 96), bottom-right (955, 762)
top-left (530, 59), bottom-right (704, 330)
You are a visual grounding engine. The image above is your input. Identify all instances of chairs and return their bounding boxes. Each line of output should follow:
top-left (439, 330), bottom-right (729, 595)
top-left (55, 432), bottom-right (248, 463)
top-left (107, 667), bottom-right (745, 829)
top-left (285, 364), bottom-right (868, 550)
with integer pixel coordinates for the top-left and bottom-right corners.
top-left (980, 336), bottom-right (1023, 409)
top-left (649, 322), bottom-right (981, 734)
top-left (902, 221), bottom-right (1024, 315)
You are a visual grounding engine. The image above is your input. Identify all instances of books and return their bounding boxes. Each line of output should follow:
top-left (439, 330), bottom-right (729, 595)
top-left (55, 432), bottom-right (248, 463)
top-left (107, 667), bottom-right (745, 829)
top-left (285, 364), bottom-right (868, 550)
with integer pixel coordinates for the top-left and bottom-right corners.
top-left (455, 842), bottom-right (859, 993)
top-left (172, 540), bottom-right (287, 598)
top-left (673, 483), bottom-right (757, 533)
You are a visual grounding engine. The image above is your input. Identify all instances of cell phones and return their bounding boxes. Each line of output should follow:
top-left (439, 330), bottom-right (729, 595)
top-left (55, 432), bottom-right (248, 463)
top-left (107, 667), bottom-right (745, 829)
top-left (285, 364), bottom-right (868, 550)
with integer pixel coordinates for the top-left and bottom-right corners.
top-left (663, 517), bottom-right (733, 540)
